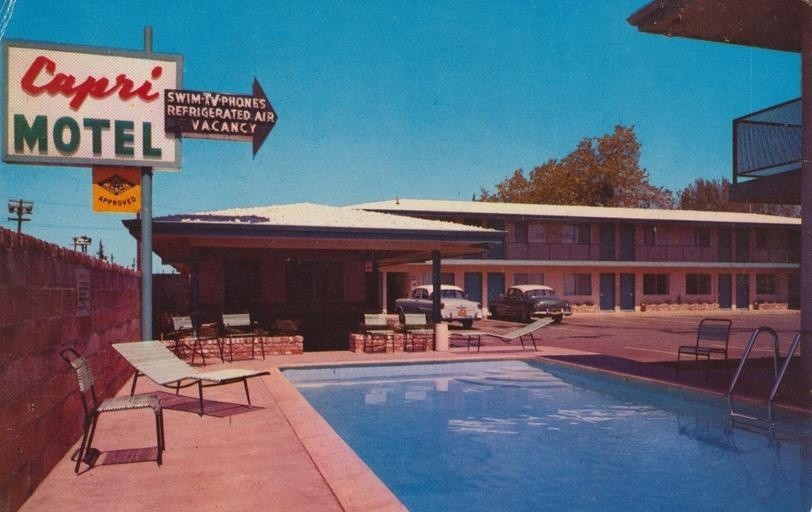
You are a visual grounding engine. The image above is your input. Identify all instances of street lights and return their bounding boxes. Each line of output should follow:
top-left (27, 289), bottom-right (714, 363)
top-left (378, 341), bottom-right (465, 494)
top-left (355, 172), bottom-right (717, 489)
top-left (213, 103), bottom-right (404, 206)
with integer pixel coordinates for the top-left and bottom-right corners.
top-left (8, 198), bottom-right (33, 233)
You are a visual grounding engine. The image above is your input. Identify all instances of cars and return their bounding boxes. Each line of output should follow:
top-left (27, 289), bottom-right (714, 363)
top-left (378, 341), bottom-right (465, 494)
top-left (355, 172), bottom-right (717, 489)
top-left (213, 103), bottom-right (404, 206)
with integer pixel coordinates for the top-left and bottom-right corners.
top-left (488, 284), bottom-right (573, 323)
top-left (395, 284), bottom-right (483, 329)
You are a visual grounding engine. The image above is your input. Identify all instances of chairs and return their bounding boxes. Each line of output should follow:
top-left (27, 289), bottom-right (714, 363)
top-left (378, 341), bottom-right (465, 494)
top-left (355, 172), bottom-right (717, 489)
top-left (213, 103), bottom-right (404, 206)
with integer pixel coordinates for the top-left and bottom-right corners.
top-left (171, 316), bottom-right (224, 366)
top-left (363, 314), bottom-right (395, 353)
top-left (59, 347), bottom-right (166, 474)
top-left (220, 314), bottom-right (266, 362)
top-left (677, 318), bottom-right (732, 381)
top-left (404, 313), bottom-right (435, 352)
top-left (448, 316), bottom-right (554, 353)
top-left (111, 340), bottom-right (271, 416)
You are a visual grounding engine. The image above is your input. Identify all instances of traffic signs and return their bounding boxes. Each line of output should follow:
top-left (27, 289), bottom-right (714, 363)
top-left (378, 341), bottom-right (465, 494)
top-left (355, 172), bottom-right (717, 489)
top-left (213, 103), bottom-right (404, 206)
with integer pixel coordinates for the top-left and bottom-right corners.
top-left (164, 76), bottom-right (278, 161)
